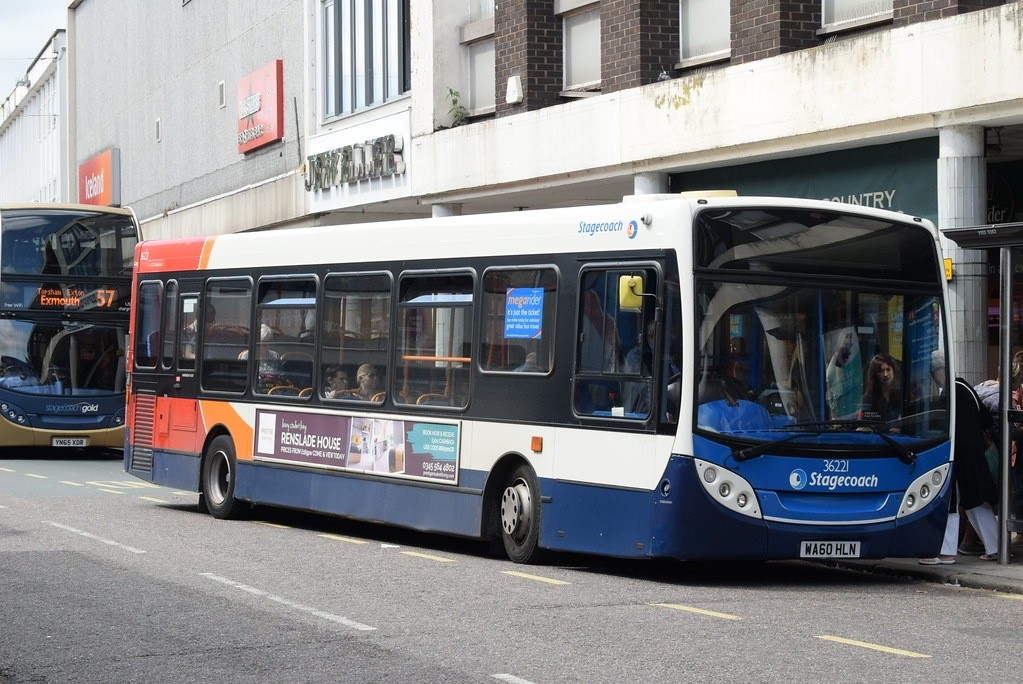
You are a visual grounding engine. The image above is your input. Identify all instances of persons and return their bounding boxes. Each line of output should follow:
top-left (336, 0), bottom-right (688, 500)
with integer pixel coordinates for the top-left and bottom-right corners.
top-left (956, 350), bottom-right (1023, 560)
top-left (858, 352), bottom-right (905, 434)
top-left (239, 323), bottom-right (297, 396)
top-left (341, 363), bottom-right (384, 402)
top-left (181, 302), bottom-right (218, 358)
top-left (919, 351), bottom-right (1010, 561)
top-left (323, 364), bottom-right (353, 401)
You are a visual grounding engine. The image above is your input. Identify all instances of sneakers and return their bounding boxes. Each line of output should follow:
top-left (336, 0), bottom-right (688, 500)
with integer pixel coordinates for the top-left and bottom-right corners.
top-left (957, 542), bottom-right (986, 555)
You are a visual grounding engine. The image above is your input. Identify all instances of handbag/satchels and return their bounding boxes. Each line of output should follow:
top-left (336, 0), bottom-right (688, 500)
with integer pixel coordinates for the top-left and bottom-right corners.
top-left (974, 430), bottom-right (999, 489)
top-left (257, 373), bottom-right (292, 395)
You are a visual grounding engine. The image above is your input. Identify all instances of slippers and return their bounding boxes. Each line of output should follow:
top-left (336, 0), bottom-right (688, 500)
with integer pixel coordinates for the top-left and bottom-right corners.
top-left (978, 553), bottom-right (1013, 561)
top-left (918, 557), bottom-right (955, 565)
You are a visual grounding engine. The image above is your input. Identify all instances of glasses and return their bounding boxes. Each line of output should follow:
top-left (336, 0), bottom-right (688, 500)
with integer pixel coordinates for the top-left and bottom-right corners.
top-left (206, 319), bottom-right (216, 325)
top-left (364, 373), bottom-right (380, 379)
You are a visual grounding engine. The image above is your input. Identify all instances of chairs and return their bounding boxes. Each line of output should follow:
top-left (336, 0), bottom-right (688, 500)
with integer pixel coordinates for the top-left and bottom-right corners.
top-left (267, 385), bottom-right (301, 396)
top-left (297, 386), bottom-right (332, 399)
top-left (148, 322), bottom-right (385, 384)
top-left (370, 391), bottom-right (408, 404)
top-left (330, 389), bottom-right (368, 400)
top-left (416, 394), bottom-right (451, 407)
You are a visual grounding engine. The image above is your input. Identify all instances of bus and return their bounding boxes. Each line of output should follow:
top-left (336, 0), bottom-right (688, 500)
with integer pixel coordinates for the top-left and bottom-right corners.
top-left (125, 190), bottom-right (956, 571)
top-left (0, 203), bottom-right (145, 448)
